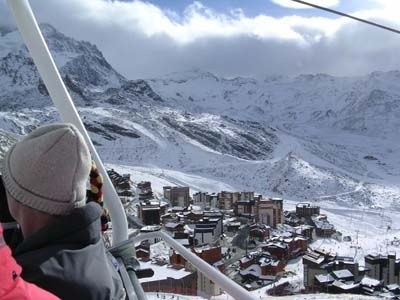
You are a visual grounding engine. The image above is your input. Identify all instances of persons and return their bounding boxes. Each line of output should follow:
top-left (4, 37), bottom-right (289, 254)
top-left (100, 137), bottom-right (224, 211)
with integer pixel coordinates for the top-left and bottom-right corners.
top-left (0, 122), bottom-right (127, 300)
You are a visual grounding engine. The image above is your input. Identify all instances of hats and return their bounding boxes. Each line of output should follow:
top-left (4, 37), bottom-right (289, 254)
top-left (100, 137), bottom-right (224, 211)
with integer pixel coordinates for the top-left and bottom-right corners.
top-left (0, 124), bottom-right (91, 214)
